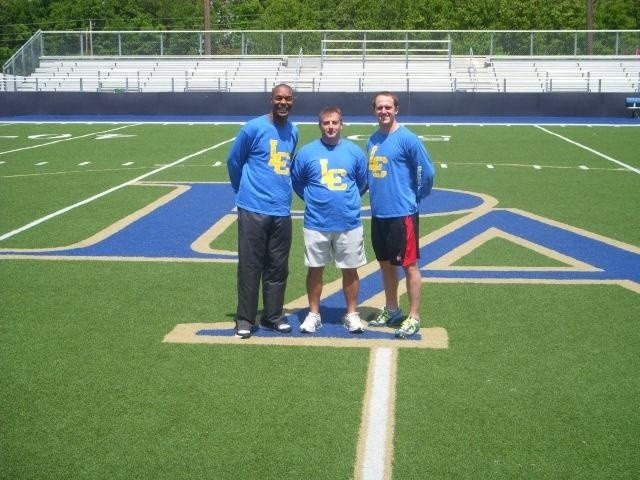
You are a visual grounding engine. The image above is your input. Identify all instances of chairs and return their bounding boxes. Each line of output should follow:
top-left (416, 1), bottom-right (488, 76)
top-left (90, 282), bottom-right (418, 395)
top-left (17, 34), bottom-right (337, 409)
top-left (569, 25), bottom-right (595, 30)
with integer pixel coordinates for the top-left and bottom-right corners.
top-left (624, 96), bottom-right (640, 118)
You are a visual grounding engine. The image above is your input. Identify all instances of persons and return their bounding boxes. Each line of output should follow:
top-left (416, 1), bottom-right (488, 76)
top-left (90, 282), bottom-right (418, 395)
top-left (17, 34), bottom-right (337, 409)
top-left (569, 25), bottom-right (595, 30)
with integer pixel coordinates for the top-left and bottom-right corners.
top-left (226, 82), bottom-right (301, 339)
top-left (359, 89), bottom-right (437, 340)
top-left (290, 105), bottom-right (371, 335)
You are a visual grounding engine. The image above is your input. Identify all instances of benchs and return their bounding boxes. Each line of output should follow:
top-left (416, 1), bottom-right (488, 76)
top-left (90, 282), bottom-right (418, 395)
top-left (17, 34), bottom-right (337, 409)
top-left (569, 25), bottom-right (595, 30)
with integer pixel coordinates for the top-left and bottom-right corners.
top-left (1, 56), bottom-right (640, 95)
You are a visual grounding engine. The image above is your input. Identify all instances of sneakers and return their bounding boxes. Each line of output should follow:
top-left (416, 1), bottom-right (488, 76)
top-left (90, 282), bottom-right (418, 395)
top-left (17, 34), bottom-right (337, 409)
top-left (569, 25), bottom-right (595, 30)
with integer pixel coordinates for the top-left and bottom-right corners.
top-left (234, 326), bottom-right (256, 339)
top-left (259, 322), bottom-right (292, 332)
top-left (368, 307), bottom-right (404, 328)
top-left (299, 311), bottom-right (323, 333)
top-left (342, 312), bottom-right (365, 334)
top-left (394, 316), bottom-right (420, 338)
